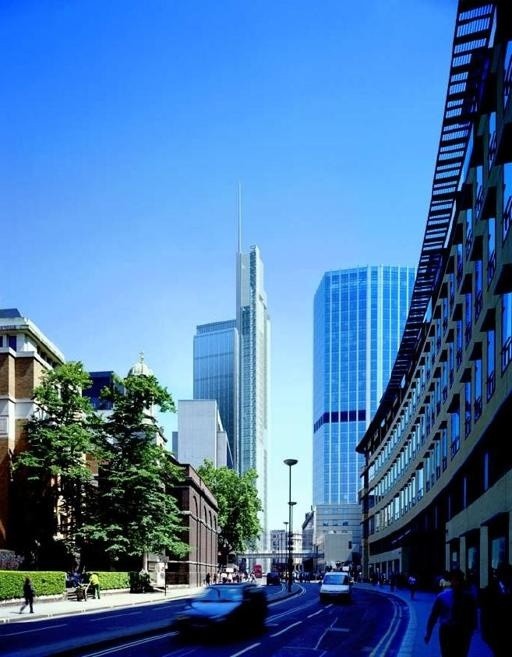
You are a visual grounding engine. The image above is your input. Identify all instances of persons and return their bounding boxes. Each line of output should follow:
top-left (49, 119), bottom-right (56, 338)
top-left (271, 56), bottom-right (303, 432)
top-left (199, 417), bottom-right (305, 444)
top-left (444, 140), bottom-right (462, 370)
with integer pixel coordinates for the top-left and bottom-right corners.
top-left (89, 573), bottom-right (100, 599)
top-left (423, 567), bottom-right (478, 657)
top-left (206, 568), bottom-right (257, 586)
top-left (138, 568), bottom-right (151, 583)
top-left (19, 577), bottom-right (37, 614)
top-left (479, 559), bottom-right (512, 657)
top-left (297, 559), bottom-right (480, 601)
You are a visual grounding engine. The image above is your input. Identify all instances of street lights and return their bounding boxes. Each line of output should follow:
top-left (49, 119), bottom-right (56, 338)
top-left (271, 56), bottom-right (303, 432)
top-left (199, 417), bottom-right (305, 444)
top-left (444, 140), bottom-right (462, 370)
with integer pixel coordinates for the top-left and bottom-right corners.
top-left (282, 457), bottom-right (299, 594)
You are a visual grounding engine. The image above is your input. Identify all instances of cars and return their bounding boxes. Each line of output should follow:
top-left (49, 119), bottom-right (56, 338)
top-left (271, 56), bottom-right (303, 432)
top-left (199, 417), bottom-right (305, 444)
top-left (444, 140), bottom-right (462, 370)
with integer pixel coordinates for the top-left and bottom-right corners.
top-left (175, 580), bottom-right (269, 647)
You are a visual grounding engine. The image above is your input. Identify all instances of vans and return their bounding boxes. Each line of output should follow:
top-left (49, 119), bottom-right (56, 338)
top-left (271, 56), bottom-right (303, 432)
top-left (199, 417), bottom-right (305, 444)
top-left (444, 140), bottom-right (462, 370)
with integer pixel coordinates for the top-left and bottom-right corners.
top-left (319, 569), bottom-right (353, 603)
